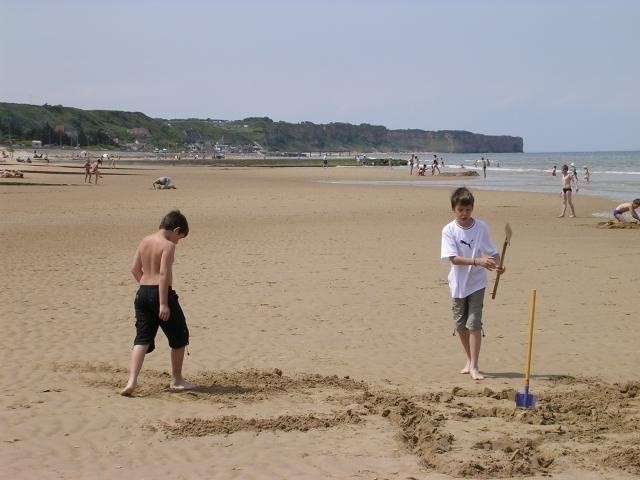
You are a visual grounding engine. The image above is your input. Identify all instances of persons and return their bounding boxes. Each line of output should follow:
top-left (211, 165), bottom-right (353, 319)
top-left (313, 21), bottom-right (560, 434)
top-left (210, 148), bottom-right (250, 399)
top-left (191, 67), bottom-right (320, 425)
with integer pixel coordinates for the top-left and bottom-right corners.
top-left (83, 149), bottom-right (122, 186)
top-left (121, 209), bottom-right (197, 397)
top-left (439, 188), bottom-right (507, 380)
top-left (569, 163), bottom-right (578, 182)
top-left (583, 166), bottom-right (589, 183)
top-left (0, 150), bottom-right (50, 178)
top-left (609, 199), bottom-right (640, 224)
top-left (357, 155), bottom-right (367, 167)
top-left (408, 154), bottom-right (500, 179)
top-left (151, 176), bottom-right (178, 190)
top-left (552, 165), bottom-right (557, 176)
top-left (557, 164), bottom-right (579, 218)
top-left (322, 153), bottom-right (329, 169)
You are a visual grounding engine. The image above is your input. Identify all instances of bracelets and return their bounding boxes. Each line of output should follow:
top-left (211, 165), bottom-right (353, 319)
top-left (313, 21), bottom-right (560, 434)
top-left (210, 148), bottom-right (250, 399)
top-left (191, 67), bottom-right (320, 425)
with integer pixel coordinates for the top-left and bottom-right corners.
top-left (473, 258), bottom-right (476, 267)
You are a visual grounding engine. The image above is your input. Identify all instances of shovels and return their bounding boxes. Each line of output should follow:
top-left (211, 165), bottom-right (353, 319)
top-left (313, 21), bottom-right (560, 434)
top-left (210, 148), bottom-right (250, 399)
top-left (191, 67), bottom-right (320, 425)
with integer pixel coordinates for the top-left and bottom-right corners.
top-left (492, 223), bottom-right (537, 408)
